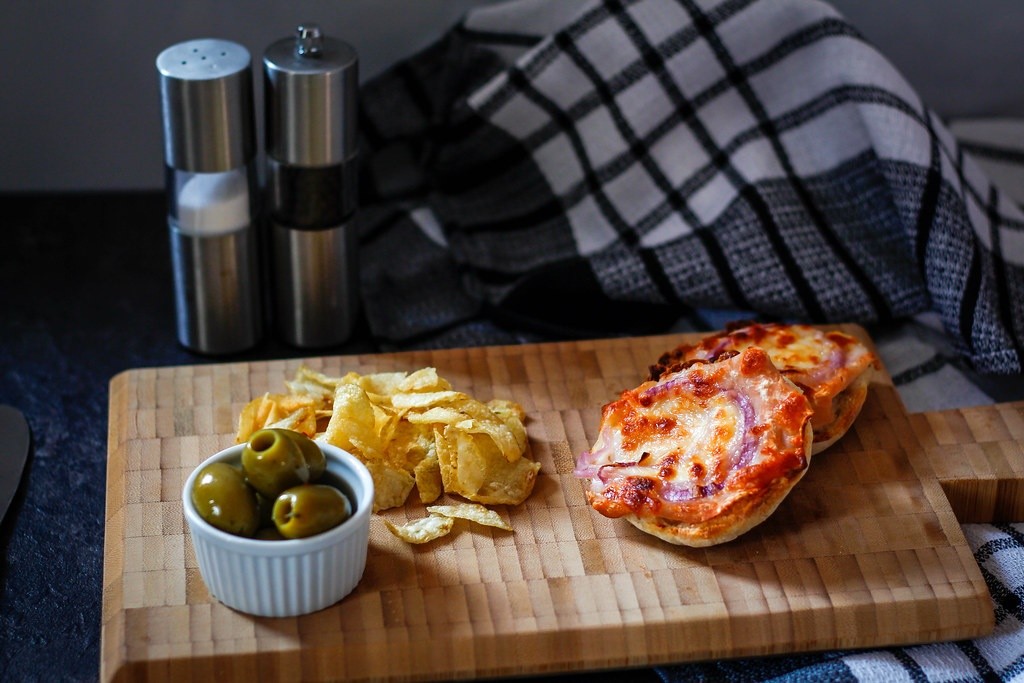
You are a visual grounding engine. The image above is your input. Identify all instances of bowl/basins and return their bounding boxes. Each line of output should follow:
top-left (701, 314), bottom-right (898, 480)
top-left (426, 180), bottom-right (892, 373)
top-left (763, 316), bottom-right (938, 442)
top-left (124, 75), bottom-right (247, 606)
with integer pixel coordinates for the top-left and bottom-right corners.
top-left (183, 435), bottom-right (376, 617)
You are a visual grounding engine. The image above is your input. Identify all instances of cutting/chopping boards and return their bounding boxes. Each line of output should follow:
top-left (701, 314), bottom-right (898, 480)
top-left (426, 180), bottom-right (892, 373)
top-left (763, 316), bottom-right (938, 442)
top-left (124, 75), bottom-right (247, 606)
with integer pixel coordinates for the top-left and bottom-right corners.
top-left (101, 323), bottom-right (1024, 682)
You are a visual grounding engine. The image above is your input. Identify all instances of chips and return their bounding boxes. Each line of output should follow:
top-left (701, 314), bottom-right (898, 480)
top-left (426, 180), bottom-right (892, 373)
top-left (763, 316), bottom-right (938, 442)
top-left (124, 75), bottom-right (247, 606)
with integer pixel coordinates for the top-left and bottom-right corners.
top-left (236, 371), bottom-right (542, 544)
